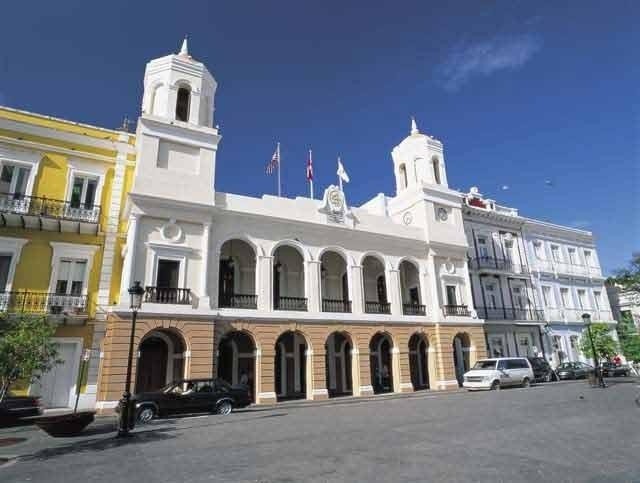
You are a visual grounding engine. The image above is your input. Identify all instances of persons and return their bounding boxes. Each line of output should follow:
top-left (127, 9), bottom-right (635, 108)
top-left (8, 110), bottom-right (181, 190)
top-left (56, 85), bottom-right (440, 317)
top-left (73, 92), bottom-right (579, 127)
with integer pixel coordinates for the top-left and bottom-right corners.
top-left (239, 370), bottom-right (248, 385)
top-left (178, 382), bottom-right (193, 395)
top-left (382, 362), bottom-right (389, 385)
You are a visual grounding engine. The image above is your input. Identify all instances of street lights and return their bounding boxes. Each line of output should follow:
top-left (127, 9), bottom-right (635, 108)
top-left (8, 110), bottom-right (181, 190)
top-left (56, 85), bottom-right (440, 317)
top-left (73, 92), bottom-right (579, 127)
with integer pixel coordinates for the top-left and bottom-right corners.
top-left (582, 312), bottom-right (606, 388)
top-left (113, 278), bottom-right (147, 437)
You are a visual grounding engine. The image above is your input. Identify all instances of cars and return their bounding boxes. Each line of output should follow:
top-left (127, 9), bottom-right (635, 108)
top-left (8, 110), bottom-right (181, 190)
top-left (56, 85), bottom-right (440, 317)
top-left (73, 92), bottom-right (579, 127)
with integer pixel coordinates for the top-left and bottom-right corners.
top-left (555, 360), bottom-right (595, 380)
top-left (599, 360), bottom-right (632, 376)
top-left (530, 356), bottom-right (553, 383)
top-left (114, 375), bottom-right (253, 424)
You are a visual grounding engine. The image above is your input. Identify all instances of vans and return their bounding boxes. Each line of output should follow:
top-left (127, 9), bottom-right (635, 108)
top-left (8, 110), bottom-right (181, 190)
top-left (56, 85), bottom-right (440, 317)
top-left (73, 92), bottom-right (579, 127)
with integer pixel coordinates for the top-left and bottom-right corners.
top-left (461, 356), bottom-right (536, 393)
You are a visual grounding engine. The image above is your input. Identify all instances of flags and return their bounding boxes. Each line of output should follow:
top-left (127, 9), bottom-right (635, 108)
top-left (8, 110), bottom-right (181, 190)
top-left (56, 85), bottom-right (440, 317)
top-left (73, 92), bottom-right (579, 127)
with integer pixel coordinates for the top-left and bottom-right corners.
top-left (306, 157), bottom-right (313, 181)
top-left (337, 159), bottom-right (350, 184)
top-left (264, 146), bottom-right (278, 175)
